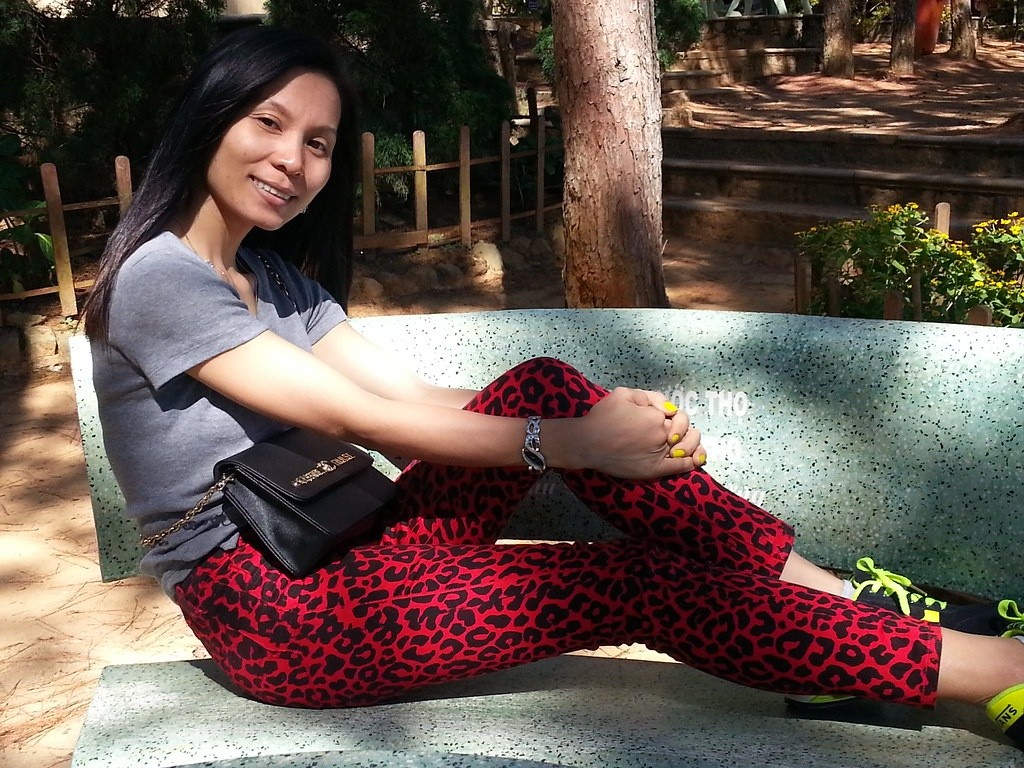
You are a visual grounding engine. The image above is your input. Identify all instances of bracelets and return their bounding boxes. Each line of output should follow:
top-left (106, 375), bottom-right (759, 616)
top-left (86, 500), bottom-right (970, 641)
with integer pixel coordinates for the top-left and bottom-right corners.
top-left (520, 415), bottom-right (546, 477)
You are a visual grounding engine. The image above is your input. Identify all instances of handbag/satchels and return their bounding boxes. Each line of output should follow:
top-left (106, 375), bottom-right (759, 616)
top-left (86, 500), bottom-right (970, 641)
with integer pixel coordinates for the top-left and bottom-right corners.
top-left (213, 427), bottom-right (409, 580)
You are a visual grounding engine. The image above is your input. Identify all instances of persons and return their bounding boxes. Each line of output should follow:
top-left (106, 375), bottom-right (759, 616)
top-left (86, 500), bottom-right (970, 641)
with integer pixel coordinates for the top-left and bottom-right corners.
top-left (72, 26), bottom-right (1024, 749)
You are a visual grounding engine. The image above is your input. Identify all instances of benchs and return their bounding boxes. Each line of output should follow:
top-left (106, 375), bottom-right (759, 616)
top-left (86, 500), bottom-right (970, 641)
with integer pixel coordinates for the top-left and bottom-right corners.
top-left (69, 308), bottom-right (1024, 768)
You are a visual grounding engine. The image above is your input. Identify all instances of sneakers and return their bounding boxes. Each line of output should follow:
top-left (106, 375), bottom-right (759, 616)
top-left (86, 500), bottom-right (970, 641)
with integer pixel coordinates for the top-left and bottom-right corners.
top-left (781, 557), bottom-right (1014, 716)
top-left (985, 599), bottom-right (1024, 741)
